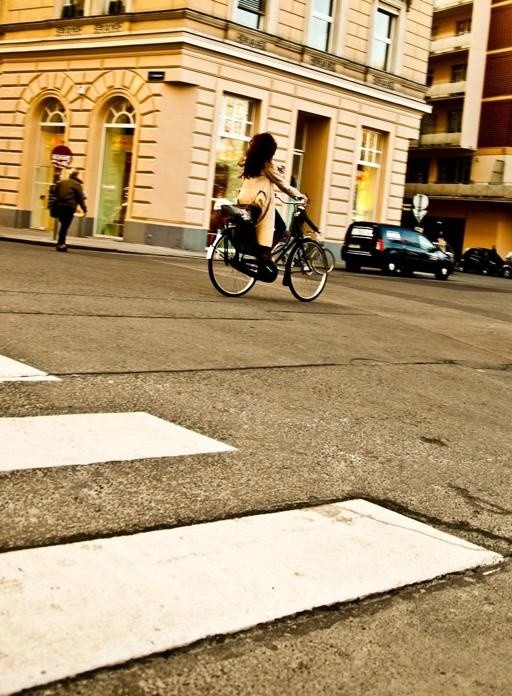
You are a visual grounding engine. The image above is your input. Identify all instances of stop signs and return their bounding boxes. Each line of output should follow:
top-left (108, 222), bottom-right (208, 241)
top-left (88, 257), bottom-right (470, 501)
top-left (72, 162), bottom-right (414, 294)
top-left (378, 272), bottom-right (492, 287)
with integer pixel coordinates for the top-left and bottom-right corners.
top-left (50, 147), bottom-right (73, 170)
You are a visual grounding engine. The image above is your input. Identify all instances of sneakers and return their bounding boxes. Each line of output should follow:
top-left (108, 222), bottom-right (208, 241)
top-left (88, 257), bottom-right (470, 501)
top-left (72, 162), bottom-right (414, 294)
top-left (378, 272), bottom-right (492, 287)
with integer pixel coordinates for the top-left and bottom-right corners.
top-left (55, 243), bottom-right (67, 253)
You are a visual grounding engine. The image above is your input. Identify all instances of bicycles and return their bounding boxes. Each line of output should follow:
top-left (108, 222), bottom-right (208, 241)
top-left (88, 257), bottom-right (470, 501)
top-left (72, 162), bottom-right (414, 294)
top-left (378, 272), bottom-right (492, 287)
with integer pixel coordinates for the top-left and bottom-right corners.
top-left (206, 194), bottom-right (335, 301)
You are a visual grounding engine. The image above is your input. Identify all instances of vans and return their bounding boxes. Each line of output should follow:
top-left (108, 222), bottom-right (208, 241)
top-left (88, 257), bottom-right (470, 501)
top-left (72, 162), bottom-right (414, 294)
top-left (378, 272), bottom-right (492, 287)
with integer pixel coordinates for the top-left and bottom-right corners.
top-left (341, 221), bottom-right (452, 280)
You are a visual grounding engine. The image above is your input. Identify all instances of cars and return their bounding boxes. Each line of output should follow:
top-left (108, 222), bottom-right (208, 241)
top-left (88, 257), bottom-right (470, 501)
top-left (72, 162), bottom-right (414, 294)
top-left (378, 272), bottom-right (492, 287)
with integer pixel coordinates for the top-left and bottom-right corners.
top-left (456, 247), bottom-right (512, 278)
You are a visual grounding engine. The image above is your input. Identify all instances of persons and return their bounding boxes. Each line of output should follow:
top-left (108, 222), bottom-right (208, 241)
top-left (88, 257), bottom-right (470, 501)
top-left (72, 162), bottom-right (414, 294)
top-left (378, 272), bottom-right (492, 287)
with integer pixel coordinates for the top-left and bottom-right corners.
top-left (289, 198), bottom-right (321, 263)
top-left (47, 168), bottom-right (87, 252)
top-left (237, 133), bottom-right (307, 256)
